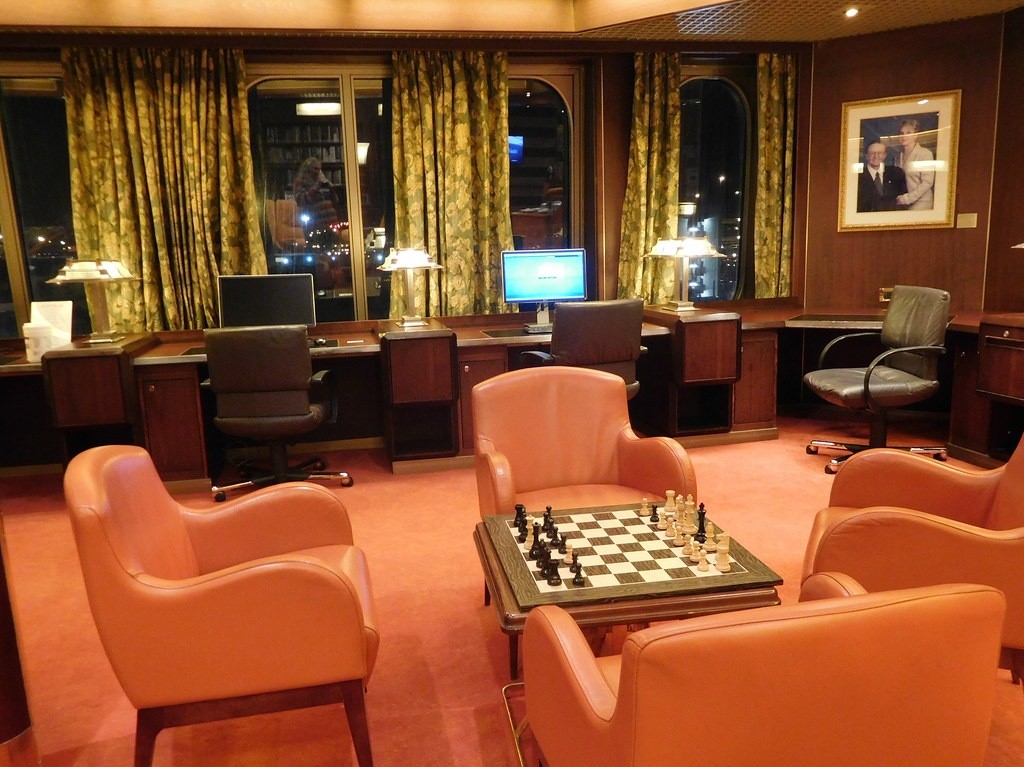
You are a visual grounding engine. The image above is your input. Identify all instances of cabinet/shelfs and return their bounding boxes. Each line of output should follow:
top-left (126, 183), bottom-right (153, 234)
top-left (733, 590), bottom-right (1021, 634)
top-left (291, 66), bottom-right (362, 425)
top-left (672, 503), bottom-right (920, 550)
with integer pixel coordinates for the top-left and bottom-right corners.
top-left (0, 304), bottom-right (1024, 480)
top-left (262, 138), bottom-right (345, 192)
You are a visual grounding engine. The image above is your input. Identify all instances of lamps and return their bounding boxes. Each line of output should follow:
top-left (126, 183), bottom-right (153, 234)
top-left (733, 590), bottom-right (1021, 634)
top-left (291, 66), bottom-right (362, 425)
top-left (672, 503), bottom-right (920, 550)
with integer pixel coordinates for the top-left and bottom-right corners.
top-left (43, 256), bottom-right (143, 344)
top-left (376, 245), bottom-right (445, 328)
top-left (640, 236), bottom-right (728, 312)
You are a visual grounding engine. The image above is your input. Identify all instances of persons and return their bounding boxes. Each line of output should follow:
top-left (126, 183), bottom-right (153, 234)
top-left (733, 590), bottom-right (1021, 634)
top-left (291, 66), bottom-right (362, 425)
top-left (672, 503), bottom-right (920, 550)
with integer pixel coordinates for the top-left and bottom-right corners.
top-left (293, 156), bottom-right (343, 286)
top-left (856, 141), bottom-right (909, 212)
top-left (889, 120), bottom-right (936, 210)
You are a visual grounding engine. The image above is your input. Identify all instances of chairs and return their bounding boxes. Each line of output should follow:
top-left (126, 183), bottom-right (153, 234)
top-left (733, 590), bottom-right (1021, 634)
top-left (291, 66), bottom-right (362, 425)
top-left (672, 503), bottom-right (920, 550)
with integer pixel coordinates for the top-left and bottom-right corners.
top-left (470, 365), bottom-right (697, 682)
top-left (201, 323), bottom-right (354, 502)
top-left (503, 570), bottom-right (1006, 767)
top-left (62, 444), bottom-right (382, 767)
top-left (802, 285), bottom-right (951, 474)
top-left (265, 198), bottom-right (306, 253)
top-left (800, 447), bottom-right (1024, 687)
top-left (519, 298), bottom-right (649, 401)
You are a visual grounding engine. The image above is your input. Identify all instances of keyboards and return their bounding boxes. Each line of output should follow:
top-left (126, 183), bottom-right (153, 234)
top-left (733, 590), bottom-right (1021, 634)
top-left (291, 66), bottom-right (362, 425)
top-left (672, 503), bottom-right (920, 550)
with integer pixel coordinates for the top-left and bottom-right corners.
top-left (523, 327), bottom-right (553, 333)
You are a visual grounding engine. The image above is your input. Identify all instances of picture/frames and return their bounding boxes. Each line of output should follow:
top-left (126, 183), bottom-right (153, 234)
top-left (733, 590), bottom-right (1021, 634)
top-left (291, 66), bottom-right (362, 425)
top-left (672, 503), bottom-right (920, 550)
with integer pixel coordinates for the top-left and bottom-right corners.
top-left (836, 89), bottom-right (962, 233)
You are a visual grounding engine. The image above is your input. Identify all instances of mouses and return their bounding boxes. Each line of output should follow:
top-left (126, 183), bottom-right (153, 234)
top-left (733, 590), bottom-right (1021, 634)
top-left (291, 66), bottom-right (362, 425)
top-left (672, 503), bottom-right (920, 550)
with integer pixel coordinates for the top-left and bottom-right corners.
top-left (314, 338), bottom-right (327, 345)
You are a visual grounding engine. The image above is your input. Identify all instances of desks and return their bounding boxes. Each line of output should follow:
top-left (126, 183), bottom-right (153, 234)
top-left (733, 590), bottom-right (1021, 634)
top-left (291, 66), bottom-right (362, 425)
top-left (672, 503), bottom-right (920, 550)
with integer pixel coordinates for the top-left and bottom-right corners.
top-left (472, 501), bottom-right (784, 682)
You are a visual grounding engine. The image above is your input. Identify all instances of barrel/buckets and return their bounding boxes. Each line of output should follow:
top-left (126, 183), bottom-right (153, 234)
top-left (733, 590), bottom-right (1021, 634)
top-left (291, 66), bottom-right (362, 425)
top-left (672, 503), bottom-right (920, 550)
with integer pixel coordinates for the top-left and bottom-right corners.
top-left (22, 323), bottom-right (51, 362)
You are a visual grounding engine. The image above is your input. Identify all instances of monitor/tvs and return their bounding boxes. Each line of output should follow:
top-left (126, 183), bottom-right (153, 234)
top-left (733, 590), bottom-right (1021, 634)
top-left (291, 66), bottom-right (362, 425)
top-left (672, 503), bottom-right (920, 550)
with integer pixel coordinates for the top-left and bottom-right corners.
top-left (508, 135), bottom-right (524, 164)
top-left (501, 248), bottom-right (587, 327)
top-left (217, 274), bottom-right (316, 328)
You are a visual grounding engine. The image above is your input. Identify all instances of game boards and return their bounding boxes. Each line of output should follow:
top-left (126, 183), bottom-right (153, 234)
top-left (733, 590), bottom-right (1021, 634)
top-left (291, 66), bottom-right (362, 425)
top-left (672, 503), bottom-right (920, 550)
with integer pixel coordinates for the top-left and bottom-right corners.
top-left (482, 501), bottom-right (784, 611)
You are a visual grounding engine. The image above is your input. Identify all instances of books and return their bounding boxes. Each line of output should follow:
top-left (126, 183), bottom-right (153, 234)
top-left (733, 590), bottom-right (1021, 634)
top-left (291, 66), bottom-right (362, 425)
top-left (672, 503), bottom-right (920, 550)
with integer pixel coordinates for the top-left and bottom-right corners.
top-left (264, 145), bottom-right (341, 164)
top-left (273, 166), bottom-right (295, 199)
top-left (324, 169), bottom-right (343, 185)
top-left (265, 124), bottom-right (341, 144)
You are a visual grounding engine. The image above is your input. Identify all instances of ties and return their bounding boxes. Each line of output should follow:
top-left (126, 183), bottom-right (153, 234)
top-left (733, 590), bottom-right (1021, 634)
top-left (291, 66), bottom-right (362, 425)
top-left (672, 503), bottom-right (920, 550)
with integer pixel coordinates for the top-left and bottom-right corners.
top-left (874, 172), bottom-right (882, 195)
